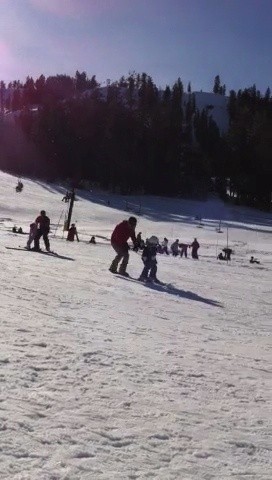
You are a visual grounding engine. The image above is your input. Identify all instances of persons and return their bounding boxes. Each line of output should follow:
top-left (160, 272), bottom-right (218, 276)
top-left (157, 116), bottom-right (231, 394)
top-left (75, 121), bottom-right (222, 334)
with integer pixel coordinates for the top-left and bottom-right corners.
top-left (222, 248), bottom-right (232, 260)
top-left (108, 216), bottom-right (140, 277)
top-left (34, 210), bottom-right (51, 252)
top-left (216, 253), bottom-right (225, 260)
top-left (170, 239), bottom-right (179, 257)
top-left (189, 237), bottom-right (199, 260)
top-left (161, 238), bottom-right (170, 255)
top-left (179, 243), bottom-right (190, 258)
top-left (26, 223), bottom-right (38, 249)
top-left (136, 232), bottom-right (142, 250)
top-left (70, 223), bottom-right (80, 242)
top-left (137, 235), bottom-right (163, 284)
top-left (249, 255), bottom-right (260, 264)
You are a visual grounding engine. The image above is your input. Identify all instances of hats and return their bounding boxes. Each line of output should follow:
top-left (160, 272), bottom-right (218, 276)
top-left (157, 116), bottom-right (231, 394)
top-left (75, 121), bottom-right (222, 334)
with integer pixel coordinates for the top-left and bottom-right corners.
top-left (148, 236), bottom-right (159, 244)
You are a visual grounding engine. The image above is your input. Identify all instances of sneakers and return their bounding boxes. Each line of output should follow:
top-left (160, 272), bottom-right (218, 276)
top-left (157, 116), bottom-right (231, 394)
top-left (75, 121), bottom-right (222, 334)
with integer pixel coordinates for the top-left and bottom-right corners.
top-left (139, 275), bottom-right (149, 281)
top-left (118, 271), bottom-right (128, 277)
top-left (150, 276), bottom-right (159, 283)
top-left (109, 268), bottom-right (118, 274)
